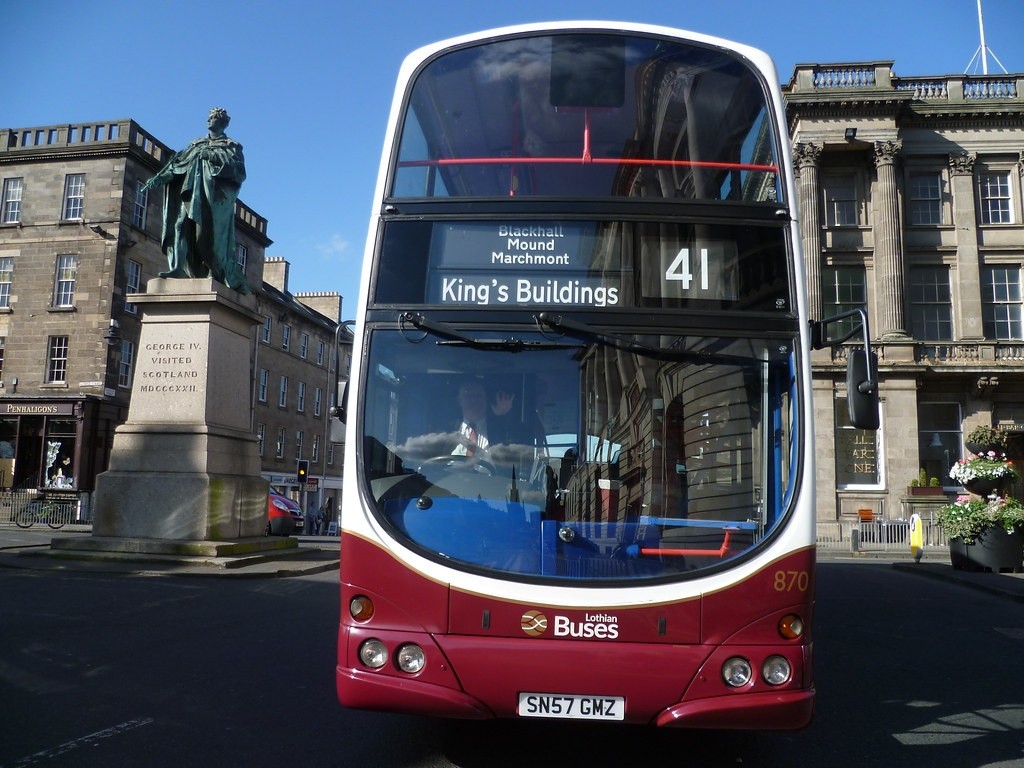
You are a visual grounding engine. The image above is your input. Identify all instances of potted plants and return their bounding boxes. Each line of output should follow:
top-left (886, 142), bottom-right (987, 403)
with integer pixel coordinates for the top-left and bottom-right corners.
top-left (963, 424), bottom-right (1008, 457)
top-left (907, 468), bottom-right (944, 496)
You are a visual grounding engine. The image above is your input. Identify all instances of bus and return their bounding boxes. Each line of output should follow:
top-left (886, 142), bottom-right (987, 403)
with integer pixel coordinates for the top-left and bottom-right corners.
top-left (333, 17), bottom-right (882, 750)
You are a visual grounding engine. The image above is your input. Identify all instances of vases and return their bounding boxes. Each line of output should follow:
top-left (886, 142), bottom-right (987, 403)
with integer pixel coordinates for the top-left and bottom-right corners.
top-left (948, 520), bottom-right (1024, 574)
top-left (958, 477), bottom-right (1017, 495)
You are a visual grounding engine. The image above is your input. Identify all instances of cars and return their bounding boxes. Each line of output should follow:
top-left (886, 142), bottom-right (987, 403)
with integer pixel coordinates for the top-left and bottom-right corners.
top-left (266, 485), bottom-right (305, 538)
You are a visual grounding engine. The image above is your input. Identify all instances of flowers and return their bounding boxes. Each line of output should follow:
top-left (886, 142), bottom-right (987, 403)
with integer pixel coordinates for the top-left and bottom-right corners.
top-left (948, 450), bottom-right (1021, 484)
top-left (936, 494), bottom-right (1024, 545)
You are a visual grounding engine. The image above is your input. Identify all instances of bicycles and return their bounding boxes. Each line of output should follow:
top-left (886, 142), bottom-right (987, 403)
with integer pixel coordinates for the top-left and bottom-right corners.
top-left (16, 496), bottom-right (67, 529)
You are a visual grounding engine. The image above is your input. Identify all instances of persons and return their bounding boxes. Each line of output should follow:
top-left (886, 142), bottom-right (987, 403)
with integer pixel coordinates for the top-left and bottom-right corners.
top-left (307, 499), bottom-right (333, 537)
top-left (145, 107), bottom-right (251, 295)
top-left (431, 383), bottom-right (516, 477)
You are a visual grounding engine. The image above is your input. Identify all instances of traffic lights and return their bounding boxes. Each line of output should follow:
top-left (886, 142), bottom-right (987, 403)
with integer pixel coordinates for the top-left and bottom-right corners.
top-left (297, 459), bottom-right (309, 485)
